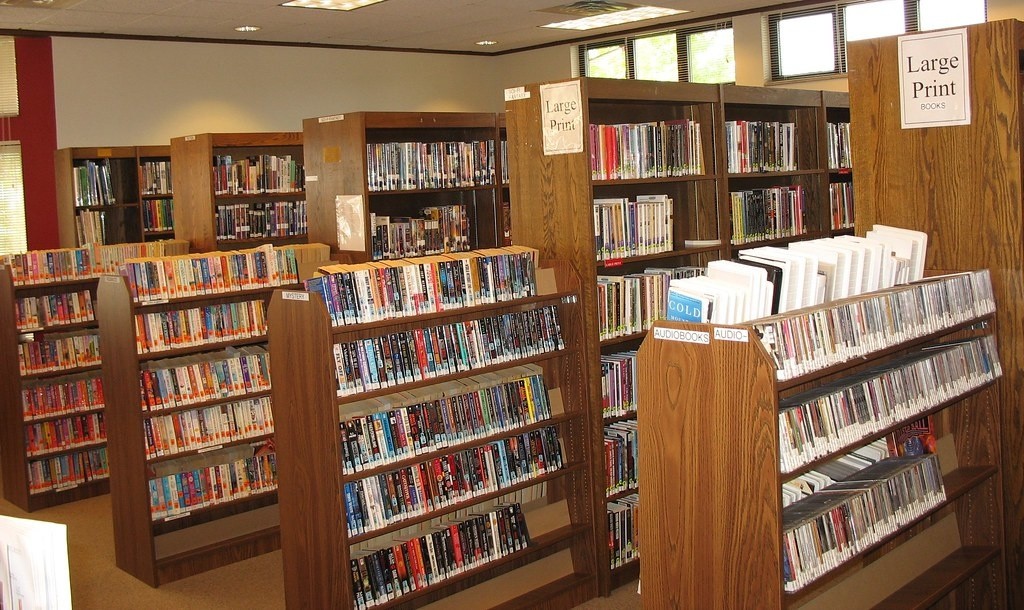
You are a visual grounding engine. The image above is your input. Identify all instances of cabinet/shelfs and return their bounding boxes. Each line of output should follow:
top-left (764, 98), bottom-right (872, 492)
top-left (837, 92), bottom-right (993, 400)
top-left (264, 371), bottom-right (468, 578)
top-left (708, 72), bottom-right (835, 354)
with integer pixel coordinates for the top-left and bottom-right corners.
top-left (845, 21), bottom-right (1024, 442)
top-left (172, 131), bottom-right (312, 251)
top-left (0, 254), bottom-right (111, 513)
top-left (301, 110), bottom-right (511, 265)
top-left (635, 272), bottom-right (1024, 610)
top-left (507, 80), bottom-right (848, 595)
top-left (96, 253), bottom-right (354, 587)
top-left (265, 260), bottom-right (597, 610)
top-left (53, 145), bottom-right (177, 246)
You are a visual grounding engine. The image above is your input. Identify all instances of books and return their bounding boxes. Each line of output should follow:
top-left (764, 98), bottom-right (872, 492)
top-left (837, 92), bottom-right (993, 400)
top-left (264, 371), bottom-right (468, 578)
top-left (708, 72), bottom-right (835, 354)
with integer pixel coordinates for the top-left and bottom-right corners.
top-left (0, 118), bottom-right (1000, 610)
